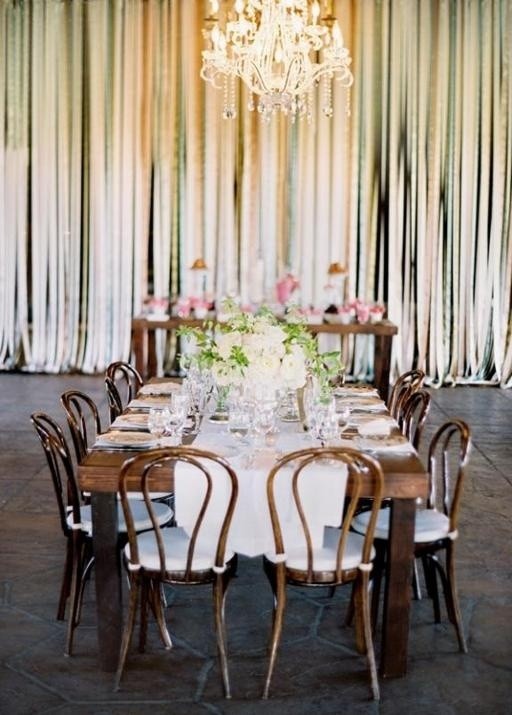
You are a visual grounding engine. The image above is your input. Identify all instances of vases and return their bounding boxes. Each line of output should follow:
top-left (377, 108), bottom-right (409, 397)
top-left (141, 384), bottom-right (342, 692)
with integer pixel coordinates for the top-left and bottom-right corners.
top-left (222, 375), bottom-right (280, 437)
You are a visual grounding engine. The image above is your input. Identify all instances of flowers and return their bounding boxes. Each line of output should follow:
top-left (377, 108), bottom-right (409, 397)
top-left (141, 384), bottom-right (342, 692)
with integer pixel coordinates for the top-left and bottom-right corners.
top-left (174, 303), bottom-right (333, 393)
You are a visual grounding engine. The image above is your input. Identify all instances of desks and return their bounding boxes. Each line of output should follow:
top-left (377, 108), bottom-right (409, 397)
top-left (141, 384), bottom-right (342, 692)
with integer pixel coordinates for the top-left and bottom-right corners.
top-left (126, 316), bottom-right (397, 399)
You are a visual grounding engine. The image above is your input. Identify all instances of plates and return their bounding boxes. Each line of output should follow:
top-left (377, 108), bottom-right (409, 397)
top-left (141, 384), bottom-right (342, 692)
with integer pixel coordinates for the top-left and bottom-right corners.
top-left (336, 395), bottom-right (386, 412)
top-left (343, 412), bottom-right (397, 430)
top-left (352, 434), bottom-right (410, 451)
top-left (97, 430), bottom-right (160, 451)
top-left (335, 384), bottom-right (380, 398)
top-left (118, 413), bottom-right (159, 426)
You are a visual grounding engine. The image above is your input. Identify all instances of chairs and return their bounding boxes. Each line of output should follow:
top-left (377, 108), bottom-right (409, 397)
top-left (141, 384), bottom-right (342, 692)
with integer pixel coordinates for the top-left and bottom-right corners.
top-left (263, 446), bottom-right (384, 703)
top-left (103, 361), bottom-right (145, 416)
top-left (113, 446), bottom-right (237, 699)
top-left (352, 421), bottom-right (472, 654)
top-left (397, 393), bottom-right (430, 440)
top-left (385, 368), bottom-right (424, 422)
top-left (30, 410), bottom-right (173, 657)
top-left (60, 390), bottom-right (172, 525)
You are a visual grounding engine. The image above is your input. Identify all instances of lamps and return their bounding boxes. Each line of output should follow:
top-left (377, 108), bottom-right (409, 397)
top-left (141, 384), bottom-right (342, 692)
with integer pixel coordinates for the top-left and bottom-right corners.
top-left (191, 259), bottom-right (210, 304)
top-left (327, 263), bottom-right (350, 311)
top-left (197, 0), bottom-right (356, 119)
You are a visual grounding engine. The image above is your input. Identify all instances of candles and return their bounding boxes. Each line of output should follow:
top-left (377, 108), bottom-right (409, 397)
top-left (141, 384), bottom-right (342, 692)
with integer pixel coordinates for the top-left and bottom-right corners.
top-left (312, 1), bottom-right (319, 26)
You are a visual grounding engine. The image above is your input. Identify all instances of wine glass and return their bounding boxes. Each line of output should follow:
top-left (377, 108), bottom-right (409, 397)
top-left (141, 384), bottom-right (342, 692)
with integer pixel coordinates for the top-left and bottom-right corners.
top-left (145, 352), bottom-right (350, 454)
